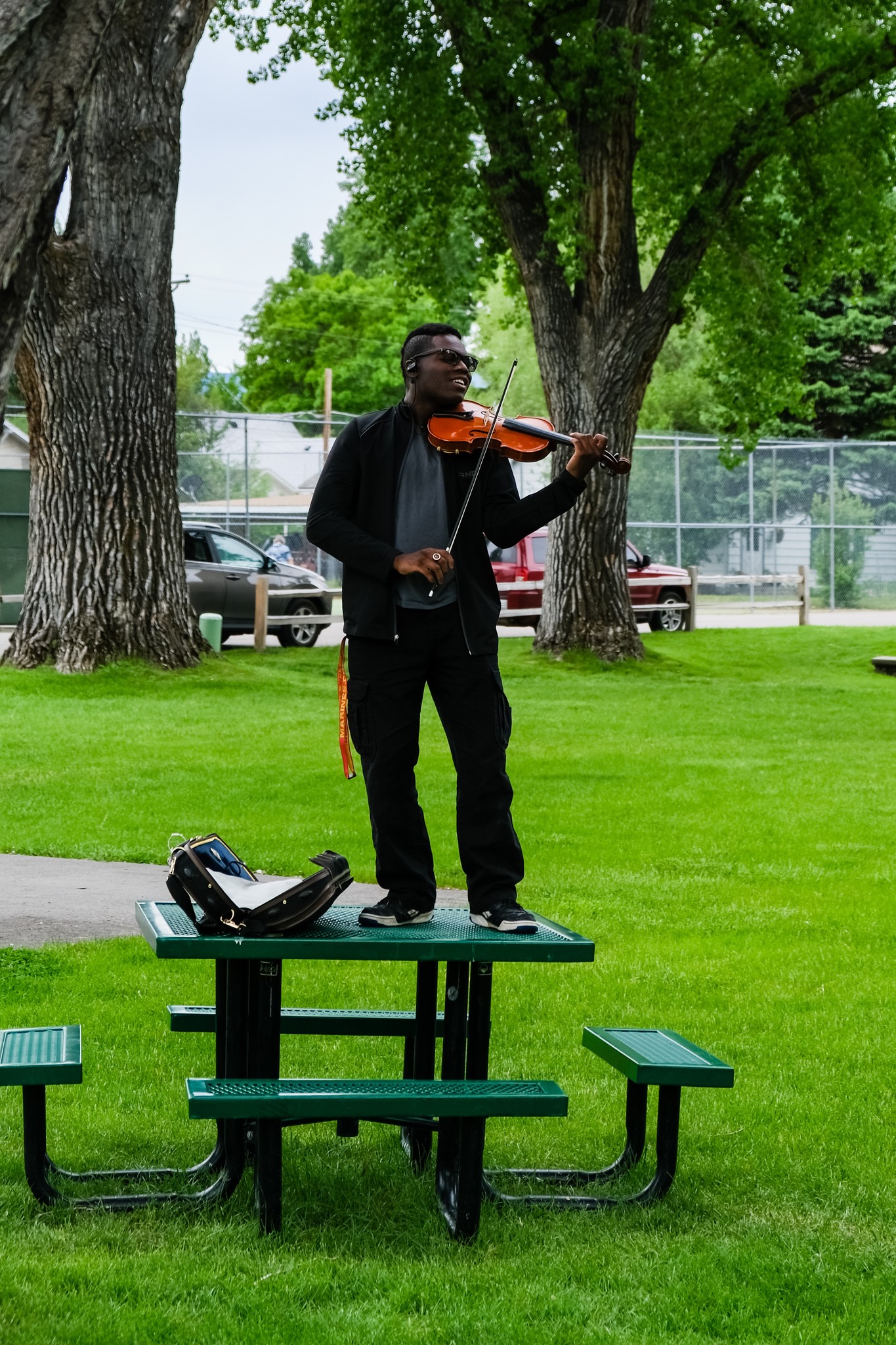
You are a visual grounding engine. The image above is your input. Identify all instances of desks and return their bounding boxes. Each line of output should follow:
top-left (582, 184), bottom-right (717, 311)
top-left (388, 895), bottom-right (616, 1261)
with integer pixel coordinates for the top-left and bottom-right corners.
top-left (25, 896), bottom-right (681, 1247)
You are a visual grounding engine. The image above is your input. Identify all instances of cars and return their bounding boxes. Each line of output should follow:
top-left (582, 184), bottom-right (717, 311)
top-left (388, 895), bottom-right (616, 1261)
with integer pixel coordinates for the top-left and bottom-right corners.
top-left (482, 524), bottom-right (692, 633)
top-left (181, 521), bottom-right (335, 649)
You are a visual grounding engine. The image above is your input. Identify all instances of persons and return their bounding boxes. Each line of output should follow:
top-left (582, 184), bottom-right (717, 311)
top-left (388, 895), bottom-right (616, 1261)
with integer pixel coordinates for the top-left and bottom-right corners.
top-left (262, 536), bottom-right (295, 564)
top-left (304, 324), bottom-right (610, 935)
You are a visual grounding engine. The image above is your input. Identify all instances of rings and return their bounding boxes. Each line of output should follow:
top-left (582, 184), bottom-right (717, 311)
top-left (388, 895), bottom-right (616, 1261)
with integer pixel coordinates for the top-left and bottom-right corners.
top-left (433, 553), bottom-right (441, 561)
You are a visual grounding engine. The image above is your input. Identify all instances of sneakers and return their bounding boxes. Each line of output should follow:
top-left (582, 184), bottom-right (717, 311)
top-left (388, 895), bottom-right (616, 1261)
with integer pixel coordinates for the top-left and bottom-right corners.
top-left (469, 898), bottom-right (538, 932)
top-left (358, 896), bottom-right (434, 927)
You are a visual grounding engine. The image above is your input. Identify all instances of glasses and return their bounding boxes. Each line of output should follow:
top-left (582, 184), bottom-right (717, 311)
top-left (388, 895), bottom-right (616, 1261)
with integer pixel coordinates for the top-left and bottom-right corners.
top-left (413, 347), bottom-right (479, 372)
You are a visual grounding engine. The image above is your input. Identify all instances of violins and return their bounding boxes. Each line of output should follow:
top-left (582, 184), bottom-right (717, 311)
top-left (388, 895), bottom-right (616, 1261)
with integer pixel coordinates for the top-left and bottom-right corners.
top-left (427, 399), bottom-right (632, 478)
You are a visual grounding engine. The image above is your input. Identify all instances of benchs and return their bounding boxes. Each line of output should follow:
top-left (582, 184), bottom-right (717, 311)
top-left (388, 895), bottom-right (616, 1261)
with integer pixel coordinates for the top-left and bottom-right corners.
top-left (183, 1077), bottom-right (569, 1126)
top-left (161, 1001), bottom-right (467, 1040)
top-left (0, 1027), bottom-right (83, 1085)
top-left (579, 1025), bottom-right (736, 1089)
